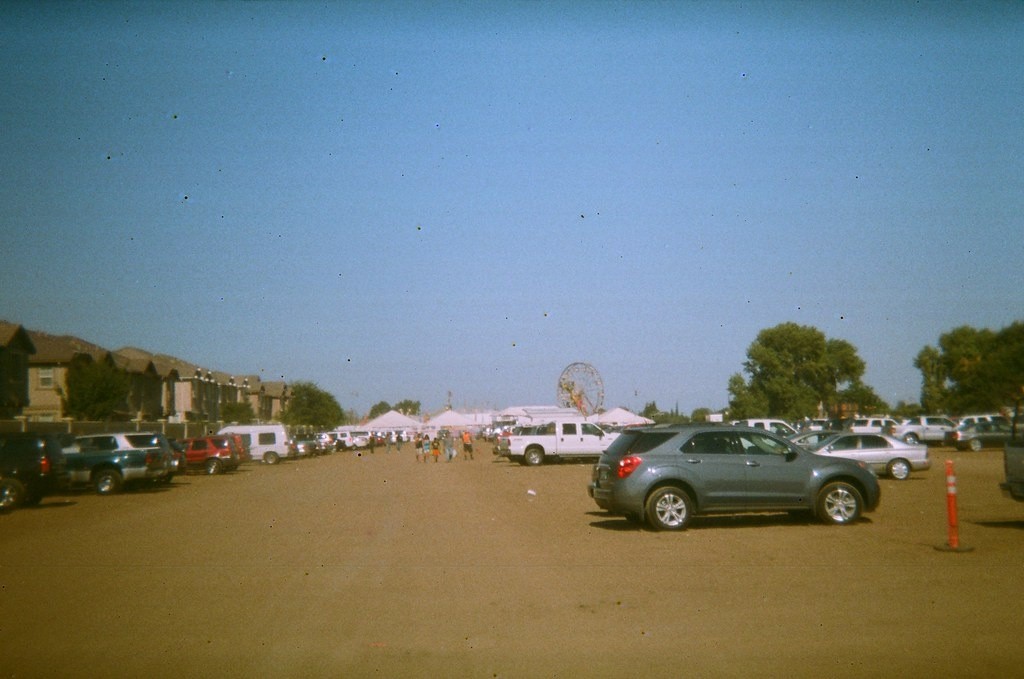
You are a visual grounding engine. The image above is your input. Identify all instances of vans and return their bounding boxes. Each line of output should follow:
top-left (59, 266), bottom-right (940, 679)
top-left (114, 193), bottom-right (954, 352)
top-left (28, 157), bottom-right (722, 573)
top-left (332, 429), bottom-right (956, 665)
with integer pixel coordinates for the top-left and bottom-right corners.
top-left (216, 424), bottom-right (294, 466)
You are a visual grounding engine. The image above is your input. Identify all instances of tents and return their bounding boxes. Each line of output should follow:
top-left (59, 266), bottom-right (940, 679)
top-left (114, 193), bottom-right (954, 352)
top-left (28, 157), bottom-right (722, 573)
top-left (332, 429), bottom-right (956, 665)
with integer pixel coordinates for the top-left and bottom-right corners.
top-left (366, 407), bottom-right (656, 428)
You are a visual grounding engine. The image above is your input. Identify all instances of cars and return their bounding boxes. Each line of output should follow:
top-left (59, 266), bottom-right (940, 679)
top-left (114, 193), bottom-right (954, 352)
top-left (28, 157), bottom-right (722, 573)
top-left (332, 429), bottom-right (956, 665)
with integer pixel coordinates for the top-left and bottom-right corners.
top-left (295, 441), bottom-right (315, 457)
top-left (803, 433), bottom-right (931, 480)
top-left (954, 414), bottom-right (1010, 426)
top-left (945, 421), bottom-right (1024, 452)
top-left (375, 436), bottom-right (384, 447)
top-left (351, 435), bottom-right (368, 451)
top-left (774, 429), bottom-right (859, 450)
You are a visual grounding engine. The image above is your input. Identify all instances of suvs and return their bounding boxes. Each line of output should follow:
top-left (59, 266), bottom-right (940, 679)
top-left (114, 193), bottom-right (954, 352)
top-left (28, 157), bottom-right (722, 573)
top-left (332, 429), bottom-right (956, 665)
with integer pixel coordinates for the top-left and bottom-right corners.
top-left (307, 433), bottom-right (333, 454)
top-left (327, 431), bottom-right (354, 452)
top-left (178, 434), bottom-right (239, 475)
top-left (0, 432), bottom-right (70, 518)
top-left (61, 432), bottom-right (164, 494)
top-left (147, 435), bottom-right (186, 489)
top-left (587, 420), bottom-right (882, 531)
top-left (224, 434), bottom-right (250, 472)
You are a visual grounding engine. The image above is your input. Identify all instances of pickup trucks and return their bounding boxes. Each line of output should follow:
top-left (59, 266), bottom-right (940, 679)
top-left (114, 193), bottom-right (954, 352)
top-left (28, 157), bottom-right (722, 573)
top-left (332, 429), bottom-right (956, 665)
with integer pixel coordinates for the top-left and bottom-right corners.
top-left (729, 419), bottom-right (802, 449)
top-left (829, 417), bottom-right (900, 434)
top-left (1000, 395), bottom-right (1024, 503)
top-left (494, 419), bottom-right (619, 467)
top-left (882, 416), bottom-right (961, 446)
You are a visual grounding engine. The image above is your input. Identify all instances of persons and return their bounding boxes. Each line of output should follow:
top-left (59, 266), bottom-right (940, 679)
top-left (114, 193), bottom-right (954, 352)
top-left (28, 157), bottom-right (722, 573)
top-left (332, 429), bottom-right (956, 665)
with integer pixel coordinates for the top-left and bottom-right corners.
top-left (369, 433), bottom-right (375, 453)
top-left (432, 438), bottom-right (440, 462)
top-left (443, 433), bottom-right (454, 461)
top-left (415, 432), bottom-right (422, 462)
top-left (422, 435), bottom-right (430, 462)
top-left (462, 431), bottom-right (476, 459)
top-left (396, 434), bottom-right (403, 453)
top-left (385, 432), bottom-right (391, 453)
